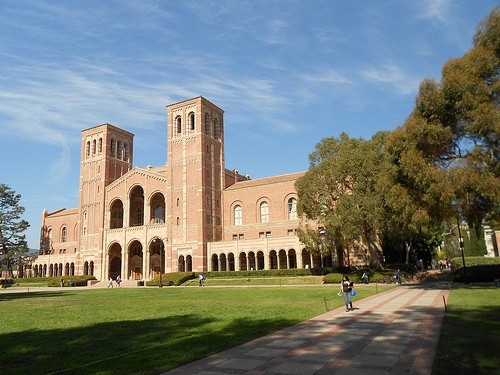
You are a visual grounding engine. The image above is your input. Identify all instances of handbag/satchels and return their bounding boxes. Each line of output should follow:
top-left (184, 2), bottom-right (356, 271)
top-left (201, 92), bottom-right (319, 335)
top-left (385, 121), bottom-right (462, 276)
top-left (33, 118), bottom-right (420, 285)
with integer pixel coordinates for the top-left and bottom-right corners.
top-left (351, 290), bottom-right (355, 296)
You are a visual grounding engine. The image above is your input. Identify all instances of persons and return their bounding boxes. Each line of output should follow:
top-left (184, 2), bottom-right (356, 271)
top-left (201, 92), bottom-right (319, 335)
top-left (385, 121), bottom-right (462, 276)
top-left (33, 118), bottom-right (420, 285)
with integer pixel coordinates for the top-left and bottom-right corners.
top-left (198, 273), bottom-right (206, 287)
top-left (431, 258), bottom-right (451, 272)
top-left (107, 277), bottom-right (113, 288)
top-left (115, 275), bottom-right (122, 288)
top-left (395, 270), bottom-right (404, 285)
top-left (338, 275), bottom-right (354, 312)
top-left (360, 271), bottom-right (370, 284)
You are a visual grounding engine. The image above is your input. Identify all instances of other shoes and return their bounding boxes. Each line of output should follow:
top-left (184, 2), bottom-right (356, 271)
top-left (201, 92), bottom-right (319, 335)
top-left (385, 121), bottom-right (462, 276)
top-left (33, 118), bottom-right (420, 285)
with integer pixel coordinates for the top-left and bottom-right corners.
top-left (346, 309), bottom-right (348, 312)
top-left (350, 308), bottom-right (353, 311)
